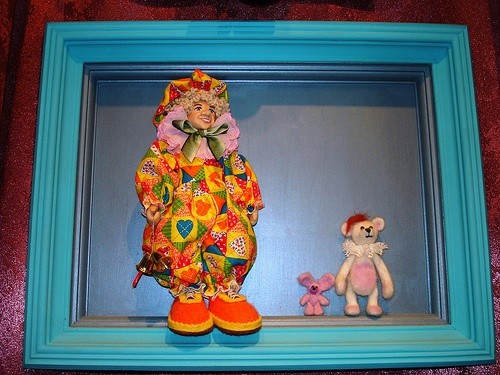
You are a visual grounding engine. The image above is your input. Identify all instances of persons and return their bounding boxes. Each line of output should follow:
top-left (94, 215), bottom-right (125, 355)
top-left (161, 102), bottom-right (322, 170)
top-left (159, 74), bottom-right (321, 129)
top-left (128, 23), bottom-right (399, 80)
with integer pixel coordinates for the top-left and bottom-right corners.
top-left (131, 67), bottom-right (264, 336)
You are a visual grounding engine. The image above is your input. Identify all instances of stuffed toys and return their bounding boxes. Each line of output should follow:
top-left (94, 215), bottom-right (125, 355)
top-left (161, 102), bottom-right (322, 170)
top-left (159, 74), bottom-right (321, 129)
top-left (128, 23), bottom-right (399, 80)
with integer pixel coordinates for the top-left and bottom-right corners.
top-left (333, 214), bottom-right (394, 317)
top-left (297, 273), bottom-right (336, 318)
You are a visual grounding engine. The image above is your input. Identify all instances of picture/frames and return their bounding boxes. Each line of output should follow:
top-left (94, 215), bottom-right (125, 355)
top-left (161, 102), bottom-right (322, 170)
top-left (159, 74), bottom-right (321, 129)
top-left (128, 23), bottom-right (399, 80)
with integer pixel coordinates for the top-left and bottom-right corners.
top-left (21, 20), bottom-right (494, 372)
